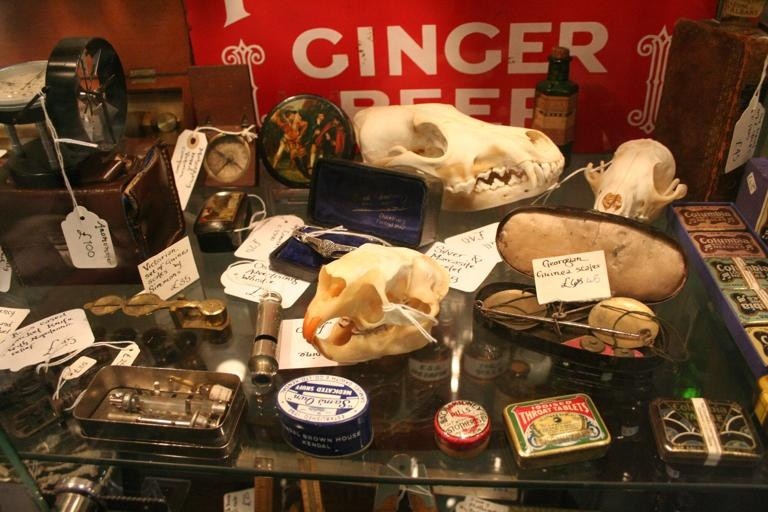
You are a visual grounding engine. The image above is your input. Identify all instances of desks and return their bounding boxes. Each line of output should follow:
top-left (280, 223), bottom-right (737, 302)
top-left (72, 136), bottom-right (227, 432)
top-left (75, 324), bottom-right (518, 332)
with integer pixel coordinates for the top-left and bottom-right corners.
top-left (0, 152), bottom-right (768, 512)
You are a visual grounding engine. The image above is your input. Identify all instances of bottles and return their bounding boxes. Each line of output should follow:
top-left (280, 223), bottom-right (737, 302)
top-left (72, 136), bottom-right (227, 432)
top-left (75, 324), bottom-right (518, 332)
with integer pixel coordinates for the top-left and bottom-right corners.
top-left (531, 47), bottom-right (579, 168)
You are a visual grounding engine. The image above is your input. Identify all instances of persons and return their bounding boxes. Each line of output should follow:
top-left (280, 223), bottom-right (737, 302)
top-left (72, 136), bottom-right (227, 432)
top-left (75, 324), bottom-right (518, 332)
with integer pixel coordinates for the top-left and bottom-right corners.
top-left (269, 99), bottom-right (345, 176)
